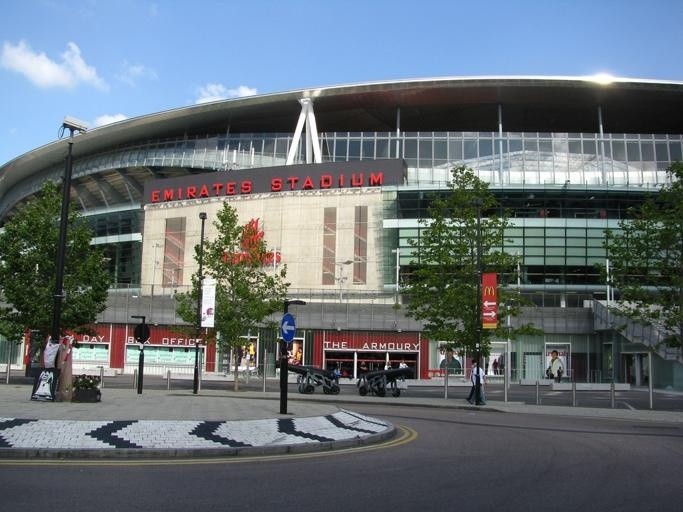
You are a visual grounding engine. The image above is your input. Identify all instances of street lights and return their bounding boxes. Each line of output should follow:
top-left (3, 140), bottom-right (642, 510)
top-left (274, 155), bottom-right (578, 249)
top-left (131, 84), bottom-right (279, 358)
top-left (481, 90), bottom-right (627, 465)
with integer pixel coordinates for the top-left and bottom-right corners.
top-left (280, 300), bottom-right (306, 414)
top-left (132, 316), bottom-right (145, 394)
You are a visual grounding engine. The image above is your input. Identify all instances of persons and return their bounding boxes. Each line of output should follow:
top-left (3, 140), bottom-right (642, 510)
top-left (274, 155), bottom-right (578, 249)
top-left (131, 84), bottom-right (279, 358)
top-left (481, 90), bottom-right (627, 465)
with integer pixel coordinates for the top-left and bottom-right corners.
top-left (356, 362), bottom-right (368, 388)
top-left (465, 359), bottom-right (488, 404)
top-left (384, 360), bottom-right (392, 370)
top-left (557, 367), bottom-right (563, 383)
top-left (398, 359), bottom-right (408, 382)
top-left (238, 337), bottom-right (257, 366)
top-left (28, 346), bottom-right (41, 364)
top-left (333, 366), bottom-right (349, 378)
top-left (644, 366), bottom-right (648, 383)
top-left (493, 358), bottom-right (504, 375)
top-left (628, 363), bottom-right (633, 384)
top-left (440, 348), bottom-right (461, 374)
top-left (550, 350), bottom-right (563, 378)
top-left (286, 347), bottom-right (302, 366)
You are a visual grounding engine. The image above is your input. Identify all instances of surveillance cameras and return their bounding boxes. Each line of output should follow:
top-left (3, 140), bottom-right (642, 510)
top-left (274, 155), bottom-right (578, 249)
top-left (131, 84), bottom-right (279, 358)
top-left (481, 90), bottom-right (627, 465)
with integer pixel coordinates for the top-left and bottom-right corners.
top-left (62, 113), bottom-right (88, 134)
